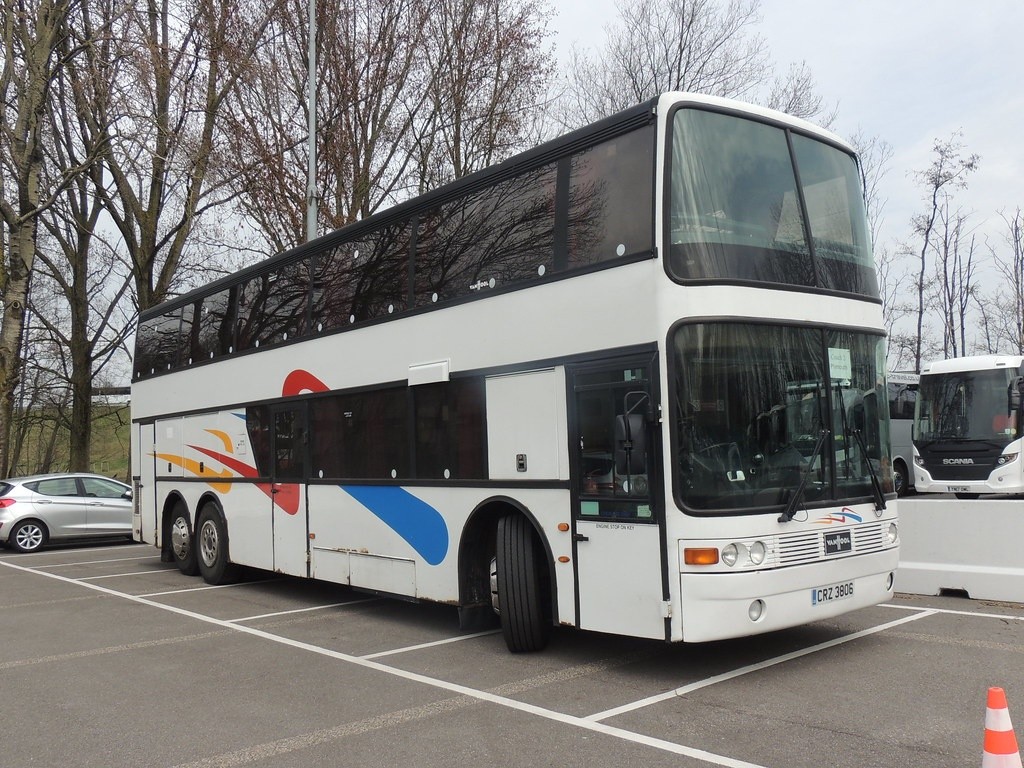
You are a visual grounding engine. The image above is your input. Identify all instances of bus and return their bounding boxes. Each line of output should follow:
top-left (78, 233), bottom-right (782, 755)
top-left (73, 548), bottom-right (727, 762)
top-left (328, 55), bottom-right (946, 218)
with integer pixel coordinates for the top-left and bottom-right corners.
top-left (911, 353), bottom-right (1024, 499)
top-left (787, 373), bottom-right (920, 497)
top-left (130, 92), bottom-right (899, 653)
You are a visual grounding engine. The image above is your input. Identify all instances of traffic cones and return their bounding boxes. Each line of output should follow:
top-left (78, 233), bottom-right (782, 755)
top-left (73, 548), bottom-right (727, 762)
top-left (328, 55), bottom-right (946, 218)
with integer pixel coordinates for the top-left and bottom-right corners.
top-left (982, 688), bottom-right (1024, 768)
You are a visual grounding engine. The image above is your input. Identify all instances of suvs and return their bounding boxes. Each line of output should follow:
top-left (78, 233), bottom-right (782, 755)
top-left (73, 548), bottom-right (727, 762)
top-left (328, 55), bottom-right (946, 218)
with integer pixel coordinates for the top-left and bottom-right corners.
top-left (0, 473), bottom-right (131, 552)
